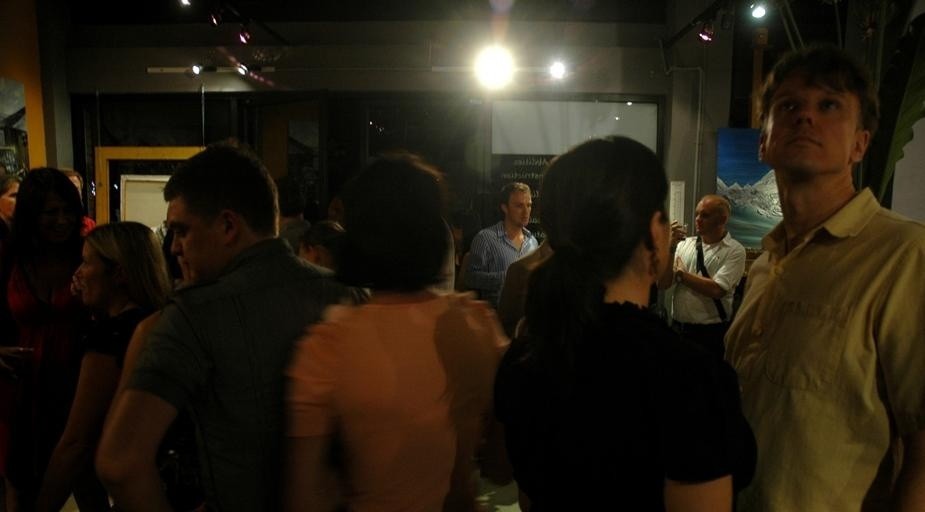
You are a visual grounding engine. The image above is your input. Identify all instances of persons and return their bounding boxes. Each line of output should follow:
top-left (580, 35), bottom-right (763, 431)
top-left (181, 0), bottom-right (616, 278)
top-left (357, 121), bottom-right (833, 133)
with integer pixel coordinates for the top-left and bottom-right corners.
top-left (458, 182), bottom-right (539, 296)
top-left (50, 222), bottom-right (170, 504)
top-left (325, 193), bottom-right (349, 226)
top-left (722, 43), bottom-right (925, 512)
top-left (0, 175), bottom-right (24, 237)
top-left (273, 172), bottom-right (308, 236)
top-left (301, 221), bottom-right (344, 265)
top-left (10, 167), bottom-right (85, 379)
top-left (657, 194), bottom-right (746, 341)
top-left (49, 168), bottom-right (84, 208)
top-left (95, 144), bottom-right (374, 512)
top-left (482, 134), bottom-right (760, 512)
top-left (287, 154), bottom-right (511, 512)
top-left (323, 194), bottom-right (346, 224)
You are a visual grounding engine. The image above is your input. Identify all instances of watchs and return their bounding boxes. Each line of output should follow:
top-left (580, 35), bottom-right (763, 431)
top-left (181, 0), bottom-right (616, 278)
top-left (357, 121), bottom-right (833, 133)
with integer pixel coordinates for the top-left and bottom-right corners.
top-left (675, 269), bottom-right (683, 285)
